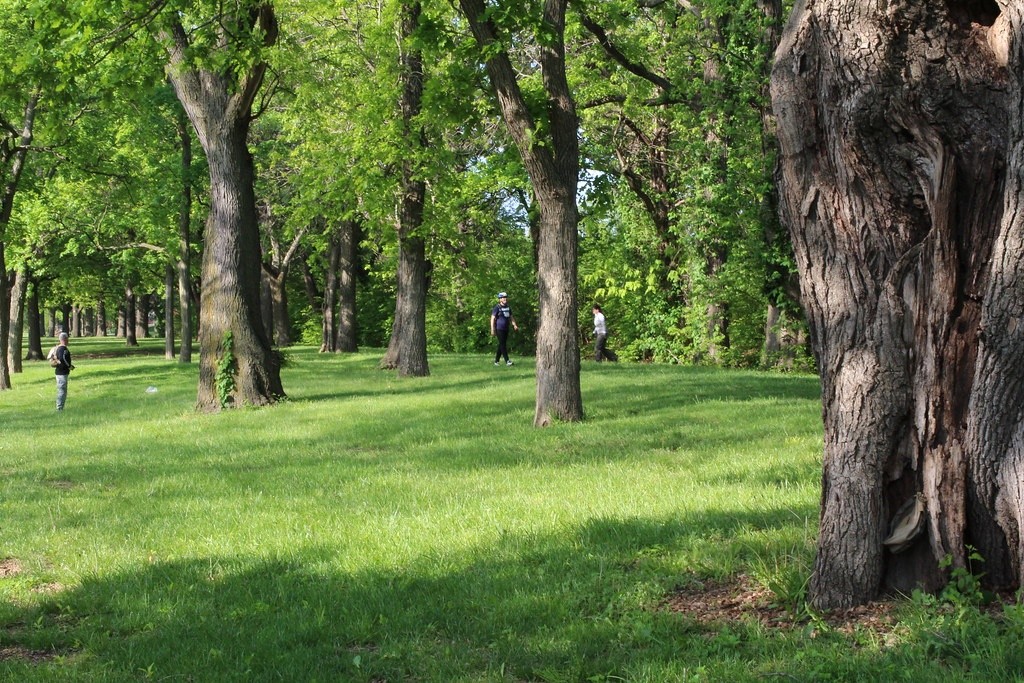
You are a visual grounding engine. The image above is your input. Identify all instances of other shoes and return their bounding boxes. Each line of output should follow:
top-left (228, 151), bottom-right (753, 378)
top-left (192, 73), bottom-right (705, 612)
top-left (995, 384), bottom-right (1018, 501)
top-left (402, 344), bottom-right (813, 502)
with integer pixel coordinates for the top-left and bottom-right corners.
top-left (494, 362), bottom-right (500, 366)
top-left (507, 360), bottom-right (514, 366)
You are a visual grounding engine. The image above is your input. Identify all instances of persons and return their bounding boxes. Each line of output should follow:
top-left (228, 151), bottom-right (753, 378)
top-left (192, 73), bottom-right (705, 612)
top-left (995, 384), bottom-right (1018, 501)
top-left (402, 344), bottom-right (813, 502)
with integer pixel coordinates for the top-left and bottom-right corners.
top-left (592, 303), bottom-right (618, 363)
top-left (54, 333), bottom-right (75, 412)
top-left (491, 291), bottom-right (519, 367)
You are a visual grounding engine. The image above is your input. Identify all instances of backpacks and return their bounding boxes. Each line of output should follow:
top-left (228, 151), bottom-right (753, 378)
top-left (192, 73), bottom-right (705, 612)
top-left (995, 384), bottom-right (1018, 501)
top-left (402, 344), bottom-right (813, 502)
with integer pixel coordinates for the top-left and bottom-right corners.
top-left (47, 345), bottom-right (65, 367)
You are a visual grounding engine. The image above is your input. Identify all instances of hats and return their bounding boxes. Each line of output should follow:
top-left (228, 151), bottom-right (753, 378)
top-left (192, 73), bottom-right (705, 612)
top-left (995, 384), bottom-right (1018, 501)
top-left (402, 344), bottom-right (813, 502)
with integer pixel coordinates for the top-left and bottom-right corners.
top-left (59, 333), bottom-right (68, 339)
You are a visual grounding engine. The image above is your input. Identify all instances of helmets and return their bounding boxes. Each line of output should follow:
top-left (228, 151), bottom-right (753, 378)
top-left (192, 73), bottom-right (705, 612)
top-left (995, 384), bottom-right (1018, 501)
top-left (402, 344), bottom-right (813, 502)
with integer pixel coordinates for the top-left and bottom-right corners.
top-left (498, 292), bottom-right (507, 298)
